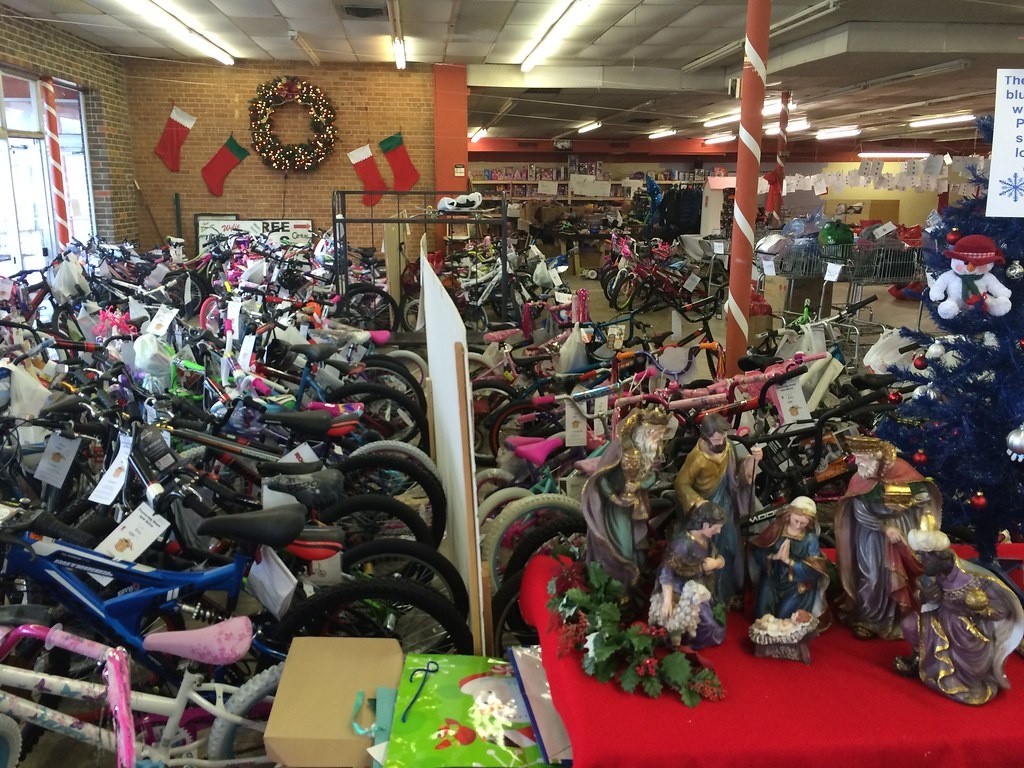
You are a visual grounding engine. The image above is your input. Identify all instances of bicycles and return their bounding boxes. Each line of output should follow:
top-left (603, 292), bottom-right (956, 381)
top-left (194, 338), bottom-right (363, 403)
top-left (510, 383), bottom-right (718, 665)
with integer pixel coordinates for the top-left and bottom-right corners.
top-left (0, 202), bottom-right (936, 768)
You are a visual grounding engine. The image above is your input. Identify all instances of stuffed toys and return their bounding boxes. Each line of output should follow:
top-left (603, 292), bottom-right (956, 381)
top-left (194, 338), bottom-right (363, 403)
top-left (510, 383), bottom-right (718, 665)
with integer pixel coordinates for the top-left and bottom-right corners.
top-left (929, 235), bottom-right (1012, 319)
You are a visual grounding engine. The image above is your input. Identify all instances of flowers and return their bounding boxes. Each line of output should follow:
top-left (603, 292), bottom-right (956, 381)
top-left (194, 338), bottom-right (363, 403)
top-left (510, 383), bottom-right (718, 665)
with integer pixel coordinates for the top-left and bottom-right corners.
top-left (539, 532), bottom-right (726, 710)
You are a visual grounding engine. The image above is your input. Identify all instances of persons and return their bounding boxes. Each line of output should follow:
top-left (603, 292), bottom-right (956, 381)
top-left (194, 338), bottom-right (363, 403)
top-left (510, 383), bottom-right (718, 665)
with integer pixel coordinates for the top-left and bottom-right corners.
top-left (580, 406), bottom-right (1010, 705)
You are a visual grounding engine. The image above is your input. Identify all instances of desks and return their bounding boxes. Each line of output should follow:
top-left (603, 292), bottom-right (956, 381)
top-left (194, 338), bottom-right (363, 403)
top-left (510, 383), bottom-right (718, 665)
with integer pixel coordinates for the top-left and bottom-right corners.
top-left (544, 225), bottom-right (638, 276)
top-left (522, 545), bottom-right (1024, 768)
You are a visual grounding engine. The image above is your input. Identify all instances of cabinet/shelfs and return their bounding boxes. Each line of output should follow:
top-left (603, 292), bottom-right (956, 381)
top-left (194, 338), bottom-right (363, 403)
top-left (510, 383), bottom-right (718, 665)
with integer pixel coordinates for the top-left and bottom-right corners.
top-left (468, 175), bottom-right (705, 217)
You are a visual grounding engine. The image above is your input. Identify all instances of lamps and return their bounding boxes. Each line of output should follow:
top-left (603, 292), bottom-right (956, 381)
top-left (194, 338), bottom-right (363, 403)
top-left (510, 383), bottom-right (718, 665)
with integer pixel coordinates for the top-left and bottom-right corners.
top-left (386, 0), bottom-right (403, 44)
top-left (483, 99), bottom-right (518, 128)
top-left (288, 31), bottom-right (320, 66)
top-left (680, 41), bottom-right (743, 75)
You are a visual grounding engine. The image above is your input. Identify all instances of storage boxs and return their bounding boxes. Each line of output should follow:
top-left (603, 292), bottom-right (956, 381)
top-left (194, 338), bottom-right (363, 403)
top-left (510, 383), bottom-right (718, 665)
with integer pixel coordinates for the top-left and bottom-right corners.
top-left (262, 637), bottom-right (404, 768)
top-left (764, 275), bottom-right (834, 330)
